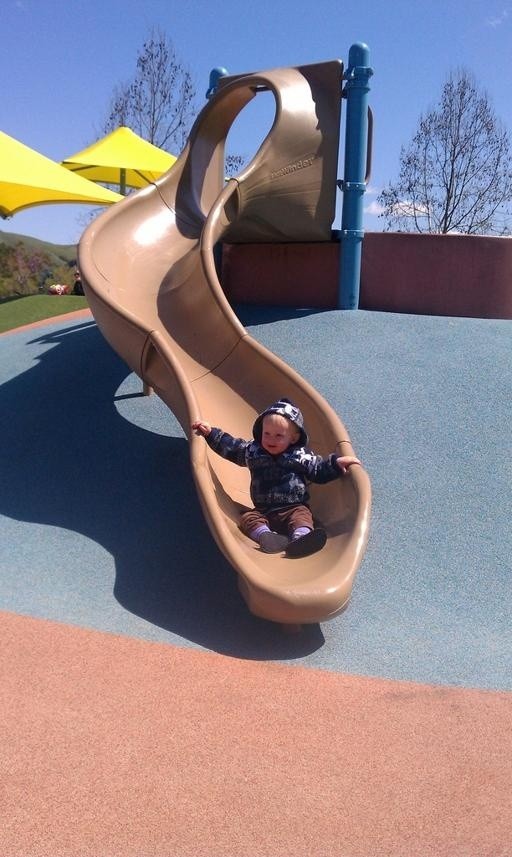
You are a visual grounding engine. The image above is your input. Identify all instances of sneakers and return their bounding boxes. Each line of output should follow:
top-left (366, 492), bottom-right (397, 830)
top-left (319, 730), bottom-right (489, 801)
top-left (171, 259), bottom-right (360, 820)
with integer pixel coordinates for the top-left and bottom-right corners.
top-left (258, 531), bottom-right (290, 555)
top-left (285, 528), bottom-right (328, 557)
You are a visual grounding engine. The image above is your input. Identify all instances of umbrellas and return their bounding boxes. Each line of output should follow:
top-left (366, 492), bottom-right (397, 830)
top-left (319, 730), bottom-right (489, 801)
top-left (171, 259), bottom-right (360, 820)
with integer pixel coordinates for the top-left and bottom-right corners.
top-left (60, 125), bottom-right (178, 195)
top-left (0, 129), bottom-right (124, 220)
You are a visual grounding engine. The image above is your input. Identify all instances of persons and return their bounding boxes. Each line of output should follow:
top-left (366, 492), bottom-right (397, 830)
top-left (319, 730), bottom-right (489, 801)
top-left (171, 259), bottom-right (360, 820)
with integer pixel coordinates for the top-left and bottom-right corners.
top-left (187, 398), bottom-right (362, 559)
top-left (70, 270), bottom-right (85, 297)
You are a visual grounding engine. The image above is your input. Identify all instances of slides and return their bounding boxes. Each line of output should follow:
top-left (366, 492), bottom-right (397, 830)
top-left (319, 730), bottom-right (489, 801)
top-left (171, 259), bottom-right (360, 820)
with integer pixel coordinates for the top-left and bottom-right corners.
top-left (76, 62), bottom-right (371, 620)
